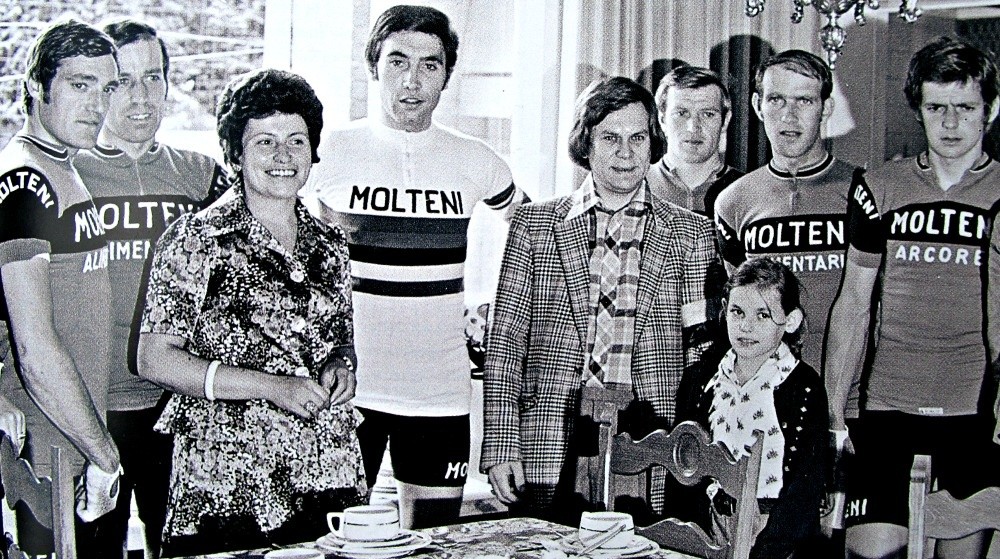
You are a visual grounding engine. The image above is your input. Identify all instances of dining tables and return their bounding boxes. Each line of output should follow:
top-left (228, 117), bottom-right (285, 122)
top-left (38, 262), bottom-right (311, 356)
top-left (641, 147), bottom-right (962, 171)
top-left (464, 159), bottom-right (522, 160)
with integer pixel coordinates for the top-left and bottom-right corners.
top-left (163, 516), bottom-right (705, 559)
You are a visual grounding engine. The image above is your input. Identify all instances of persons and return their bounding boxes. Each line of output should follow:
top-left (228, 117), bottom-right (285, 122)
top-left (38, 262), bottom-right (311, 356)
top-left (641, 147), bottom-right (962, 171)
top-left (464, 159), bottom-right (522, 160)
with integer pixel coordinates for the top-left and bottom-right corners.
top-left (297, 5), bottom-right (531, 530)
top-left (479, 32), bottom-right (1000, 559)
top-left (137, 70), bottom-right (369, 559)
top-left (0, 21), bottom-right (235, 559)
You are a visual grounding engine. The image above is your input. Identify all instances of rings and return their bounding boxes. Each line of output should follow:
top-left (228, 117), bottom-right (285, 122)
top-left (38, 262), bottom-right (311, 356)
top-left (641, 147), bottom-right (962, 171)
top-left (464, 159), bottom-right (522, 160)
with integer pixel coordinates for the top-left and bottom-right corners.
top-left (18, 435), bottom-right (26, 439)
top-left (307, 402), bottom-right (315, 411)
top-left (491, 489), bottom-right (496, 497)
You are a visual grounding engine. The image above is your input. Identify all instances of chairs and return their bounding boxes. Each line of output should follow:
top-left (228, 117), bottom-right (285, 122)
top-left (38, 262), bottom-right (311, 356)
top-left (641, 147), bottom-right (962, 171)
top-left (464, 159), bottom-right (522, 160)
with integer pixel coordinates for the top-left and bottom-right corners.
top-left (594, 397), bottom-right (766, 559)
top-left (907, 453), bottom-right (1000, 559)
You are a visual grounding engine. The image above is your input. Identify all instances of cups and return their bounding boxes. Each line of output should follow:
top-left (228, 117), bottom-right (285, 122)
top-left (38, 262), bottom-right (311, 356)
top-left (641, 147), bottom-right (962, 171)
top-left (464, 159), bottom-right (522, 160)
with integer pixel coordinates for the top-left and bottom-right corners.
top-left (327, 505), bottom-right (399, 542)
top-left (579, 512), bottom-right (634, 549)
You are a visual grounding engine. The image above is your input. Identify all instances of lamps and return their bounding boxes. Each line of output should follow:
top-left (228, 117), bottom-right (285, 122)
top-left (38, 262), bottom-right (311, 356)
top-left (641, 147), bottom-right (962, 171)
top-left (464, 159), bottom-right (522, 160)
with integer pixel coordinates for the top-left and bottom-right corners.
top-left (746, 0), bottom-right (923, 71)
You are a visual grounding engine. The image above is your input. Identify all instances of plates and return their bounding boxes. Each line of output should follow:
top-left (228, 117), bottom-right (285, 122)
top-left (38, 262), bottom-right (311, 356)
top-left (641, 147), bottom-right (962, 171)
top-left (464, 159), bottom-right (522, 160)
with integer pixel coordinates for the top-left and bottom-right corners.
top-left (555, 533), bottom-right (659, 559)
top-left (316, 530), bottom-right (432, 558)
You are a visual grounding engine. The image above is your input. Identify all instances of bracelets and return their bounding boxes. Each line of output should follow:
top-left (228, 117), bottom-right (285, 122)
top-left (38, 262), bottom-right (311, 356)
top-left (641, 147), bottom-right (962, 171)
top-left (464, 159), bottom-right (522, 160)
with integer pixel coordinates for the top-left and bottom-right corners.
top-left (337, 355), bottom-right (354, 370)
top-left (204, 360), bottom-right (221, 401)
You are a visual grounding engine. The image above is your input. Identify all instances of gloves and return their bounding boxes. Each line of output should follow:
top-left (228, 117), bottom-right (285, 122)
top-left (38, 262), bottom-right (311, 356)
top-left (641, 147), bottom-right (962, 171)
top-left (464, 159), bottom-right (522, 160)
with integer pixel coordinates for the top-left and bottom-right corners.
top-left (76, 460), bottom-right (124, 523)
